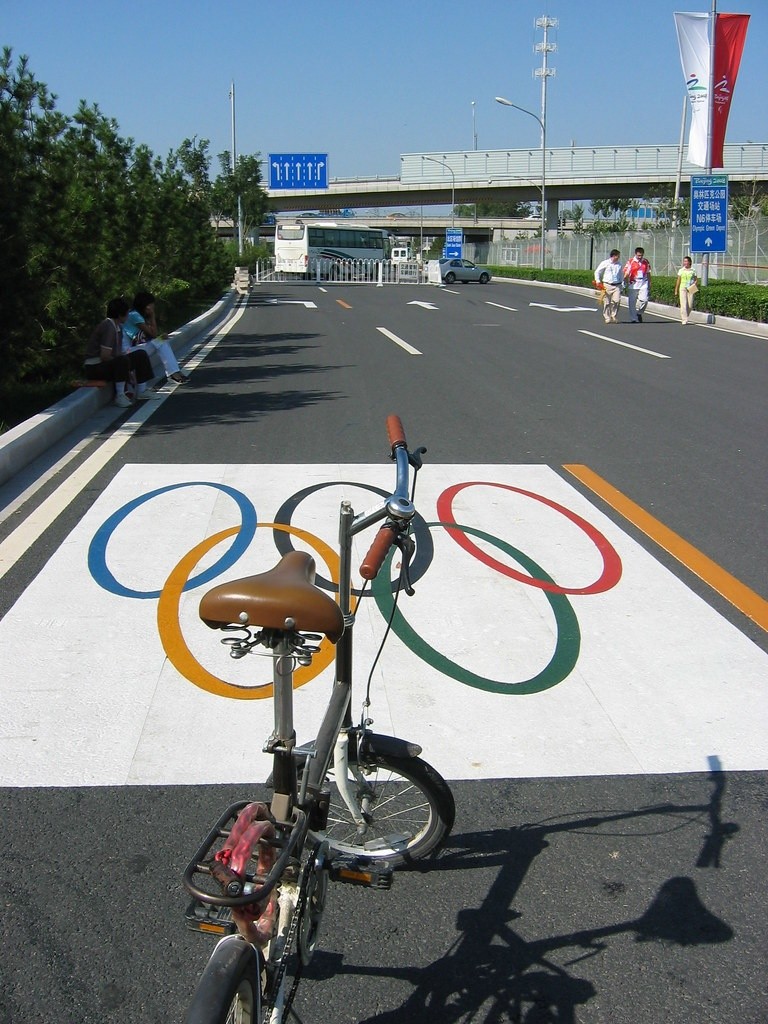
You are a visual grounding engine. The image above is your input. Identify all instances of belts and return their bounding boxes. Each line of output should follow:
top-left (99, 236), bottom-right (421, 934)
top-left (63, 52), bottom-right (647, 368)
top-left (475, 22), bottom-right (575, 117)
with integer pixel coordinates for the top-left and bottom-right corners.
top-left (606, 283), bottom-right (621, 286)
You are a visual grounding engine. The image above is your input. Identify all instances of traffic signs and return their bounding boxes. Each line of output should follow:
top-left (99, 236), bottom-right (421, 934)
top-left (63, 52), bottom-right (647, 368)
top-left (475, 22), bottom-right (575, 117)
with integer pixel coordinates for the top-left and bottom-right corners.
top-left (446, 228), bottom-right (463, 260)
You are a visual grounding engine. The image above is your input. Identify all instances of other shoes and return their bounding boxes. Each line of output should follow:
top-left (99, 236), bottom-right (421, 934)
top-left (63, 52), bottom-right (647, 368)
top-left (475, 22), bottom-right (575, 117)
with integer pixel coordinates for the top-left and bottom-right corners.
top-left (637, 313), bottom-right (643, 323)
top-left (632, 321), bottom-right (636, 323)
top-left (681, 321), bottom-right (687, 325)
top-left (137, 388), bottom-right (161, 401)
top-left (113, 394), bottom-right (132, 408)
top-left (605, 320), bottom-right (610, 324)
top-left (612, 319), bottom-right (619, 323)
top-left (167, 375), bottom-right (192, 385)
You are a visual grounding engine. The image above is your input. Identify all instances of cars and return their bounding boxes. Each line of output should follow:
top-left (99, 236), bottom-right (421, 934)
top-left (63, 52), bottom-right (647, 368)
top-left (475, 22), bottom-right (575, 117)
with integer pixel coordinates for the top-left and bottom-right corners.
top-left (424, 257), bottom-right (492, 284)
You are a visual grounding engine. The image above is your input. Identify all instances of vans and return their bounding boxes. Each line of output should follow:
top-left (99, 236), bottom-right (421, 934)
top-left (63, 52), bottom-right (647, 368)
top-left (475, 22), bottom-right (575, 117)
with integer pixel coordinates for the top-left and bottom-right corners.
top-left (391, 247), bottom-right (413, 269)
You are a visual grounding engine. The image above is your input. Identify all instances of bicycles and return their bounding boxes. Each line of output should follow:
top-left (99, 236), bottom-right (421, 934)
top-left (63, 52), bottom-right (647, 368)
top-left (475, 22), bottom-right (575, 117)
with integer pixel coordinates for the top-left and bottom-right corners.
top-left (178, 412), bottom-right (457, 1022)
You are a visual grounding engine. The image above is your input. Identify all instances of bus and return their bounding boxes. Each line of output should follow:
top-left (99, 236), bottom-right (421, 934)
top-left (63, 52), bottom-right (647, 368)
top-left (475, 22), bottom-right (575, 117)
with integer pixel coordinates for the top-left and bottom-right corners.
top-left (274, 222), bottom-right (397, 280)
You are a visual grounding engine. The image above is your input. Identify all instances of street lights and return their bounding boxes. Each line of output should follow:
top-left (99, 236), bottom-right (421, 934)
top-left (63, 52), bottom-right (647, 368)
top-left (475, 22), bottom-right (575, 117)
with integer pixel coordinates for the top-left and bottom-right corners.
top-left (493, 96), bottom-right (548, 273)
top-left (471, 101), bottom-right (479, 151)
top-left (422, 156), bottom-right (455, 228)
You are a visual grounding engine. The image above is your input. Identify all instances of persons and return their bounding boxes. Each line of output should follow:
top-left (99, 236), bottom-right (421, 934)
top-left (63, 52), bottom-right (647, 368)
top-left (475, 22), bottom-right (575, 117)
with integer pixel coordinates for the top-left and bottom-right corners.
top-left (122, 292), bottom-right (192, 384)
top-left (595, 249), bottom-right (626, 324)
top-left (675, 257), bottom-right (697, 325)
top-left (84, 299), bottom-right (161, 408)
top-left (623, 247), bottom-right (651, 323)
